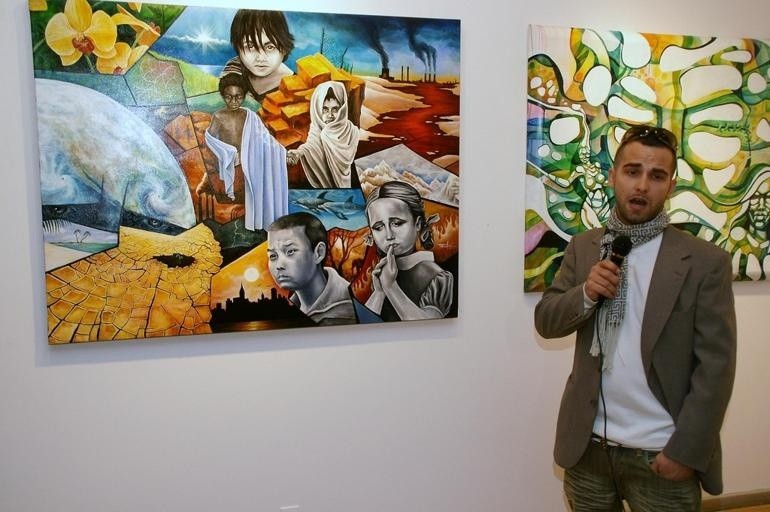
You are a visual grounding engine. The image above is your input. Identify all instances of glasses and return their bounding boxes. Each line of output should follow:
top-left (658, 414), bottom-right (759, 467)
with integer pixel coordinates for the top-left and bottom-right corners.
top-left (620, 125), bottom-right (678, 152)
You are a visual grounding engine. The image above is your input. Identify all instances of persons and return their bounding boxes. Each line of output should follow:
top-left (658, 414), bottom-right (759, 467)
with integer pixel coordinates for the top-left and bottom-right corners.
top-left (205, 72), bottom-right (288, 232)
top-left (363, 182), bottom-right (454, 320)
top-left (282, 78), bottom-right (361, 189)
top-left (221, 7), bottom-right (298, 96)
top-left (264, 209), bottom-right (358, 325)
top-left (533, 126), bottom-right (737, 510)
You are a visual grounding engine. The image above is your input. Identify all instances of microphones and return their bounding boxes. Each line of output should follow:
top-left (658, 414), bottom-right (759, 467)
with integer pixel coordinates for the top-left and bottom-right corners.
top-left (597, 235), bottom-right (633, 310)
